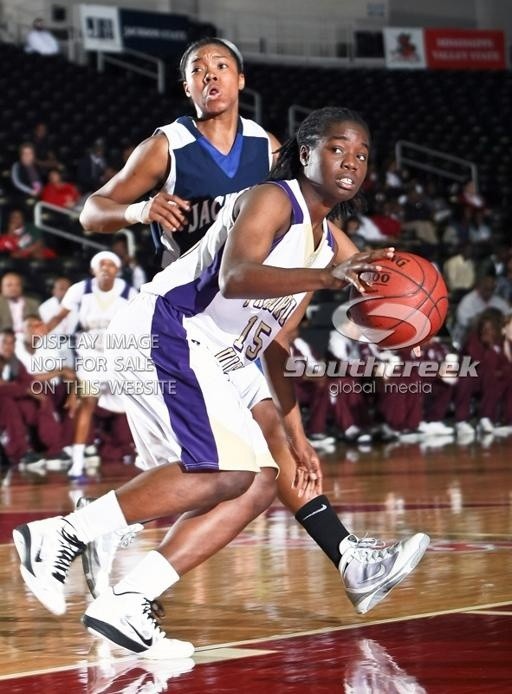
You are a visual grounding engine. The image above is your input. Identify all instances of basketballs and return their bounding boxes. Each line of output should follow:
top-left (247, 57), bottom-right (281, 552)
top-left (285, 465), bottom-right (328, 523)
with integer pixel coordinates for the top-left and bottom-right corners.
top-left (348, 250), bottom-right (448, 350)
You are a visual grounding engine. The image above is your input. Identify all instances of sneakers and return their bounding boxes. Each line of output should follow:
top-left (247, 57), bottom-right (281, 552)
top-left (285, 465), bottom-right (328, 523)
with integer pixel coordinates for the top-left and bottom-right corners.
top-left (12, 515), bottom-right (86, 615)
top-left (76, 496), bottom-right (144, 599)
top-left (307, 417), bottom-right (512, 447)
top-left (80, 585), bottom-right (195, 659)
top-left (338, 533), bottom-right (430, 614)
top-left (1, 444), bottom-right (100, 479)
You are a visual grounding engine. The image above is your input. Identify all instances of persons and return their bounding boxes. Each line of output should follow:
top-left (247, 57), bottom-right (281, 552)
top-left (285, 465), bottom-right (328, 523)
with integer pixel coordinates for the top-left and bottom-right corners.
top-left (1, 123), bottom-right (511, 484)
top-left (10, 104), bottom-right (397, 657)
top-left (24, 18), bottom-right (60, 56)
top-left (79, 37), bottom-right (429, 614)
